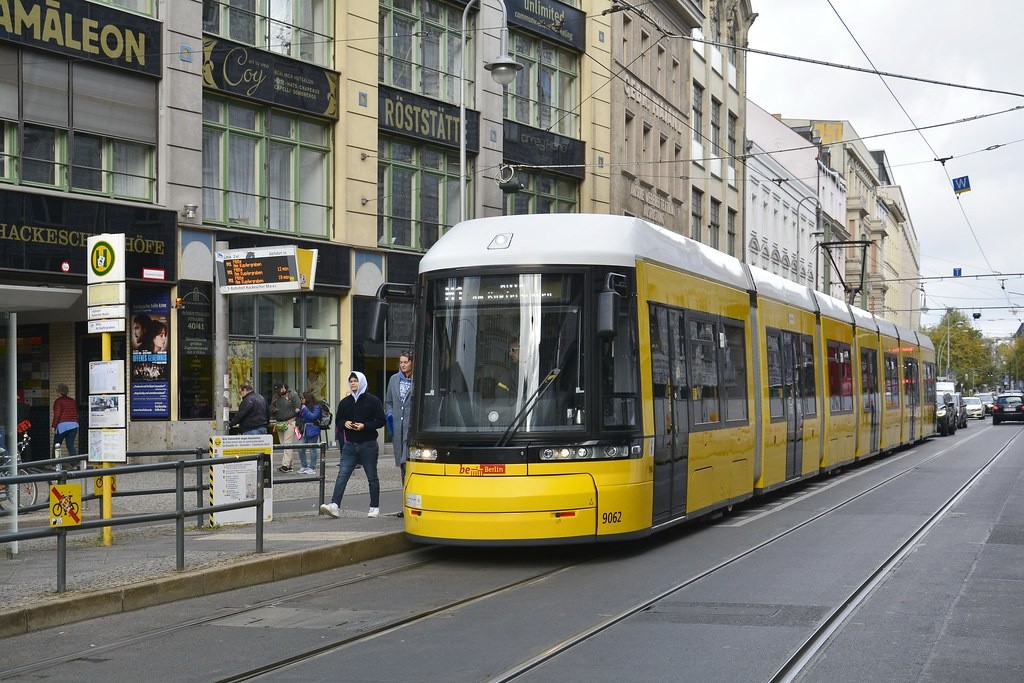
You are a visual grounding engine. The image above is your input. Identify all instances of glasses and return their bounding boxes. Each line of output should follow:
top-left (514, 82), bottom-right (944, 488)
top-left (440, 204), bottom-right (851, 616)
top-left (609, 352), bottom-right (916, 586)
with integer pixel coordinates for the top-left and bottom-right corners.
top-left (509, 348), bottom-right (518, 353)
top-left (238, 389), bottom-right (244, 394)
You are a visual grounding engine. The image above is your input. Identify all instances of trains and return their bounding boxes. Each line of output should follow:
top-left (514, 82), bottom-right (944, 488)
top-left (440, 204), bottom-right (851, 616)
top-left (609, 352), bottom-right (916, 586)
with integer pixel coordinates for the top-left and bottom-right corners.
top-left (362, 213), bottom-right (934, 546)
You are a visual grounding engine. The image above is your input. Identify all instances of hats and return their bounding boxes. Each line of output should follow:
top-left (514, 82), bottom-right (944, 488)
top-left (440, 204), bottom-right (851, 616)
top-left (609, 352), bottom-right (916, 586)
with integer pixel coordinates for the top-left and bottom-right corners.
top-left (273, 383), bottom-right (283, 394)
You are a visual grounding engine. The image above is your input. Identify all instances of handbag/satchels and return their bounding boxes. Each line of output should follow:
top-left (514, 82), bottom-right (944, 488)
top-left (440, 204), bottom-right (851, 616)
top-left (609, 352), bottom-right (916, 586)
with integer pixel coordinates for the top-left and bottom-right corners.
top-left (276, 422), bottom-right (288, 432)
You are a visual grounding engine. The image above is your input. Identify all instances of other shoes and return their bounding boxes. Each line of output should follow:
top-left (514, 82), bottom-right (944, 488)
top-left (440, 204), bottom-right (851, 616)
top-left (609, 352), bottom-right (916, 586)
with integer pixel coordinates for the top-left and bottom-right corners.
top-left (397, 511), bottom-right (403, 518)
top-left (304, 468), bottom-right (316, 474)
top-left (288, 468), bottom-right (293, 472)
top-left (277, 467), bottom-right (289, 473)
top-left (297, 467), bottom-right (309, 474)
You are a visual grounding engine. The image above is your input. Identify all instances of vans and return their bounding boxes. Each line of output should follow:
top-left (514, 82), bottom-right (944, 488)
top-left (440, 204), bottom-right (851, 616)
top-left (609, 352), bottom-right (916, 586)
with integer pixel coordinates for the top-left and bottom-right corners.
top-left (936, 382), bottom-right (955, 394)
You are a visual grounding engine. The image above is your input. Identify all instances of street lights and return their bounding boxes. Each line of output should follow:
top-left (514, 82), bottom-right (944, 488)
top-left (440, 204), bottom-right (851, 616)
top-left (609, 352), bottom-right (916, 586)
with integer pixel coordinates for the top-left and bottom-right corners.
top-left (459, 1), bottom-right (524, 221)
top-left (797, 196), bottom-right (834, 286)
top-left (910, 288), bottom-right (929, 331)
top-left (937, 321), bottom-right (969, 377)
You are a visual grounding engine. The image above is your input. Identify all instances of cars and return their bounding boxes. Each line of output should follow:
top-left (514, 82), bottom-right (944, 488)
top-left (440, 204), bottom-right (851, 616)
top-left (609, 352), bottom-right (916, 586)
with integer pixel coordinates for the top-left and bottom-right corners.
top-left (935, 392), bottom-right (957, 436)
top-left (992, 393), bottom-right (1024, 425)
top-left (961, 396), bottom-right (985, 420)
top-left (949, 392), bottom-right (968, 429)
top-left (973, 390), bottom-right (1024, 416)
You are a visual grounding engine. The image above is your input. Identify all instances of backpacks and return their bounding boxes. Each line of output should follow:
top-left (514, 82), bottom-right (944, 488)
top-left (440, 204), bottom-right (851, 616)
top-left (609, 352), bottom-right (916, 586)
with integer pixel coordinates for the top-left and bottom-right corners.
top-left (311, 399), bottom-right (333, 431)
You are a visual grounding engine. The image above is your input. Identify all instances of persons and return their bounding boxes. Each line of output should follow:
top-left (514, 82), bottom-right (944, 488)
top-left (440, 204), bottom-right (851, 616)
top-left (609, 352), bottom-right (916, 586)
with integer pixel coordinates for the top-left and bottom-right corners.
top-left (320, 371), bottom-right (387, 518)
top-left (229, 384), bottom-right (270, 434)
top-left (133, 313), bottom-right (167, 354)
top-left (507, 337), bottom-right (553, 391)
top-left (270, 381), bottom-right (302, 472)
top-left (384, 350), bottom-right (413, 518)
top-left (44, 384), bottom-right (79, 471)
top-left (295, 392), bottom-right (322, 475)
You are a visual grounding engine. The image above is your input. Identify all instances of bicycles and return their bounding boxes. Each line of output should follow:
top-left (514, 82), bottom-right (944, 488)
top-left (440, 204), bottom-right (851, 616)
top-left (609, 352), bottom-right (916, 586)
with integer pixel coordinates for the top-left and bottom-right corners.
top-left (0, 431), bottom-right (52, 515)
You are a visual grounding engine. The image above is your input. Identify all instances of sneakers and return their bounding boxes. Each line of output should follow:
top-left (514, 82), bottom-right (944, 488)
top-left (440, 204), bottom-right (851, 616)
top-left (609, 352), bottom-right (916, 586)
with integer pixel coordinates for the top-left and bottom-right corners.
top-left (320, 502), bottom-right (339, 519)
top-left (367, 506), bottom-right (380, 518)
